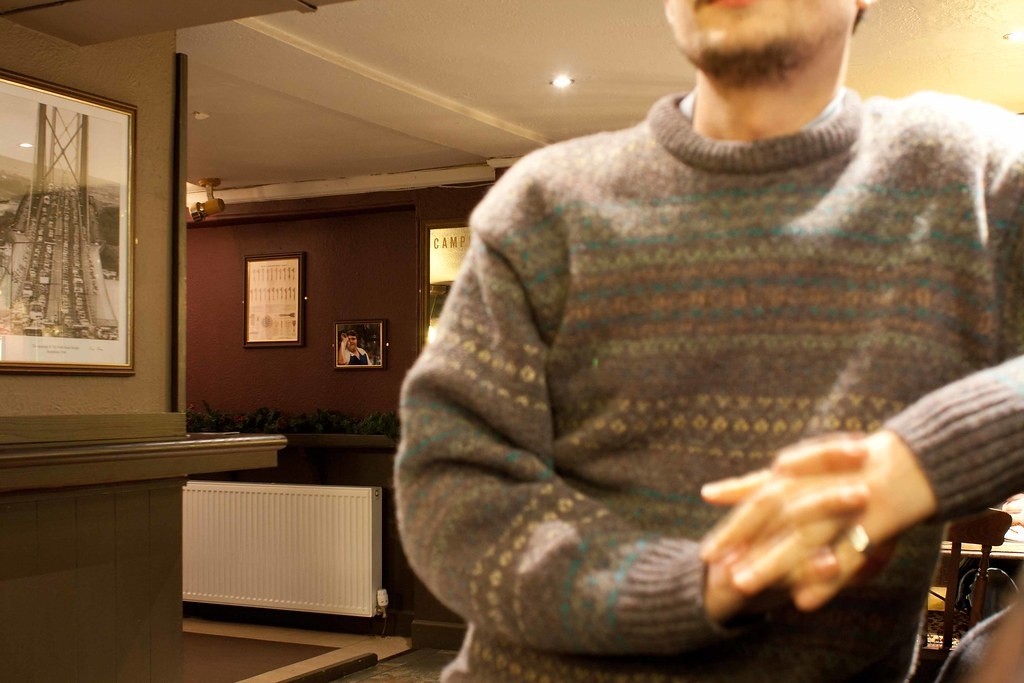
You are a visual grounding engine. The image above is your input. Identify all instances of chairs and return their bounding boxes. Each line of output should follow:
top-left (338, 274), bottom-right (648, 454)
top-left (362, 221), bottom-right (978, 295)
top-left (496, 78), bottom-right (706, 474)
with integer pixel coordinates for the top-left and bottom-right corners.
top-left (921, 508), bottom-right (1013, 662)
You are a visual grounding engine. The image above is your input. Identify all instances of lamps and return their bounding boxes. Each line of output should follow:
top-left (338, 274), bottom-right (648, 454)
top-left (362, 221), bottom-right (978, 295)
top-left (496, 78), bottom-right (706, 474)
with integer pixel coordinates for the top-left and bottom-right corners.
top-left (188, 181), bottom-right (225, 222)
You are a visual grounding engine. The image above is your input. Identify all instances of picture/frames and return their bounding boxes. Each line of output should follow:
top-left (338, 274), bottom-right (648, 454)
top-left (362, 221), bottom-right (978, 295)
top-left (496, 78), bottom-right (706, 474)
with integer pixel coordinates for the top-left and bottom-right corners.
top-left (242, 249), bottom-right (308, 349)
top-left (419, 218), bottom-right (469, 356)
top-left (333, 321), bottom-right (387, 370)
top-left (0, 69), bottom-right (139, 377)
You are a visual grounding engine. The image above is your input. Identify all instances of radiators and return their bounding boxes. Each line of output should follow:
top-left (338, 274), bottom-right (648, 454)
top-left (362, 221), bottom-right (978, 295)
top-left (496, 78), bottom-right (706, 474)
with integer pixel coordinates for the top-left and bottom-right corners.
top-left (184, 481), bottom-right (387, 620)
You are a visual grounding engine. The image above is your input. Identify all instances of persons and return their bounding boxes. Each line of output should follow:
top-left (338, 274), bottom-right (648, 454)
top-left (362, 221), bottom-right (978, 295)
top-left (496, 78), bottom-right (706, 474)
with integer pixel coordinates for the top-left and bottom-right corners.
top-left (338, 328), bottom-right (374, 365)
top-left (395, 0), bottom-right (1024, 683)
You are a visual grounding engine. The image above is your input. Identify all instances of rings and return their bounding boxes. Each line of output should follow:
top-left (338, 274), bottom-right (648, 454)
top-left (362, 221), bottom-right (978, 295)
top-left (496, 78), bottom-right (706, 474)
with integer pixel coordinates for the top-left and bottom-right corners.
top-left (848, 524), bottom-right (869, 553)
